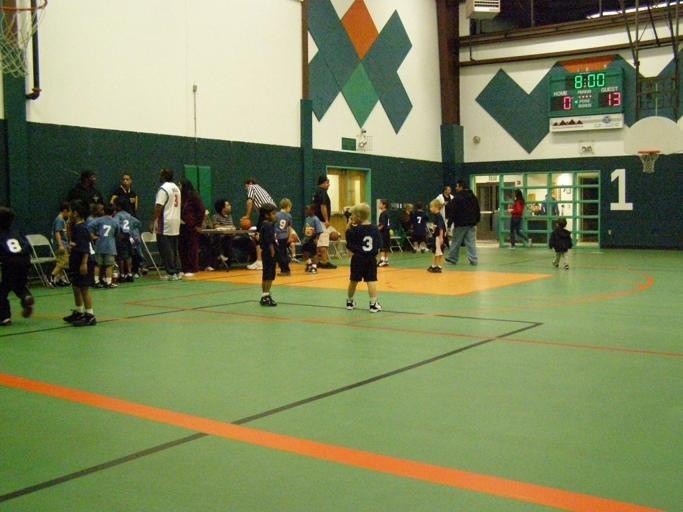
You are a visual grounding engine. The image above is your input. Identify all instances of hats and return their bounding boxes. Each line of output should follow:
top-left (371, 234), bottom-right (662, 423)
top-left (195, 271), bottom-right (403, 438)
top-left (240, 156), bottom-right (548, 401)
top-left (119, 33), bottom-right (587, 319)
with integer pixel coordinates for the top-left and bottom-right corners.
top-left (317, 177), bottom-right (327, 184)
top-left (80, 170), bottom-right (95, 181)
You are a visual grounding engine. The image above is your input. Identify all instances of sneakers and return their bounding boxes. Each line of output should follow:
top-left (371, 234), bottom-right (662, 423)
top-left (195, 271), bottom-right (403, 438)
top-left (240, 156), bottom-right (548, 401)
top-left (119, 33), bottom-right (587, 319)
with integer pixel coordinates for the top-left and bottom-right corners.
top-left (23, 295), bottom-right (34, 317)
top-left (0, 318), bottom-right (11, 326)
top-left (247, 260), bottom-right (263, 270)
top-left (45, 273), bottom-right (139, 287)
top-left (369, 301), bottom-right (382, 312)
top-left (347, 299), bottom-right (356, 310)
top-left (63, 310), bottom-right (97, 326)
top-left (306, 261), bottom-right (337, 273)
top-left (376, 260), bottom-right (388, 267)
top-left (428, 265), bottom-right (442, 273)
top-left (261, 295), bottom-right (276, 306)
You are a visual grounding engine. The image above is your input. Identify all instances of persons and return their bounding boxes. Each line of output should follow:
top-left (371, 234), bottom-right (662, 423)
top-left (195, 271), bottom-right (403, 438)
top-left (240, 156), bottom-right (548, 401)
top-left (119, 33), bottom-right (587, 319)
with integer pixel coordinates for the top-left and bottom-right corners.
top-left (376, 198), bottom-right (431, 267)
top-left (148, 168), bottom-right (205, 280)
top-left (508, 189), bottom-right (532, 249)
top-left (47, 171), bottom-right (148, 289)
top-left (549, 217), bottom-right (572, 269)
top-left (198, 177), bottom-right (324, 276)
top-left (541, 194), bottom-right (559, 216)
top-left (256, 203), bottom-right (277, 307)
top-left (1, 206), bottom-right (35, 328)
top-left (426, 179), bottom-right (480, 273)
top-left (62, 199), bottom-right (98, 327)
top-left (345, 202), bottom-right (384, 313)
top-left (310, 176), bottom-right (337, 270)
